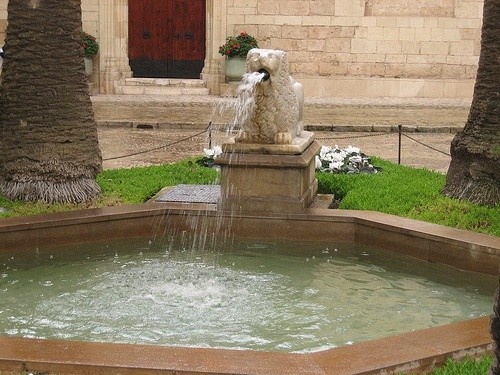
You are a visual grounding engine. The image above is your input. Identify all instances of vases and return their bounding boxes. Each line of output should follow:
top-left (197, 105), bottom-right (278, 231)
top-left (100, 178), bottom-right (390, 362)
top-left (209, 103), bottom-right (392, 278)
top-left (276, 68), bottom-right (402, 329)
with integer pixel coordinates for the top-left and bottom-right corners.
top-left (84, 58), bottom-right (93, 77)
top-left (225, 56), bottom-right (248, 83)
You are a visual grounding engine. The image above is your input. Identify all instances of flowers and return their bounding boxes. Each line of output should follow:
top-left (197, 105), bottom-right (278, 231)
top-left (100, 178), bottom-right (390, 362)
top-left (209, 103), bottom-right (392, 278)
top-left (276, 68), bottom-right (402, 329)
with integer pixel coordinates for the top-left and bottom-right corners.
top-left (81, 32), bottom-right (100, 59)
top-left (217, 31), bottom-right (260, 58)
